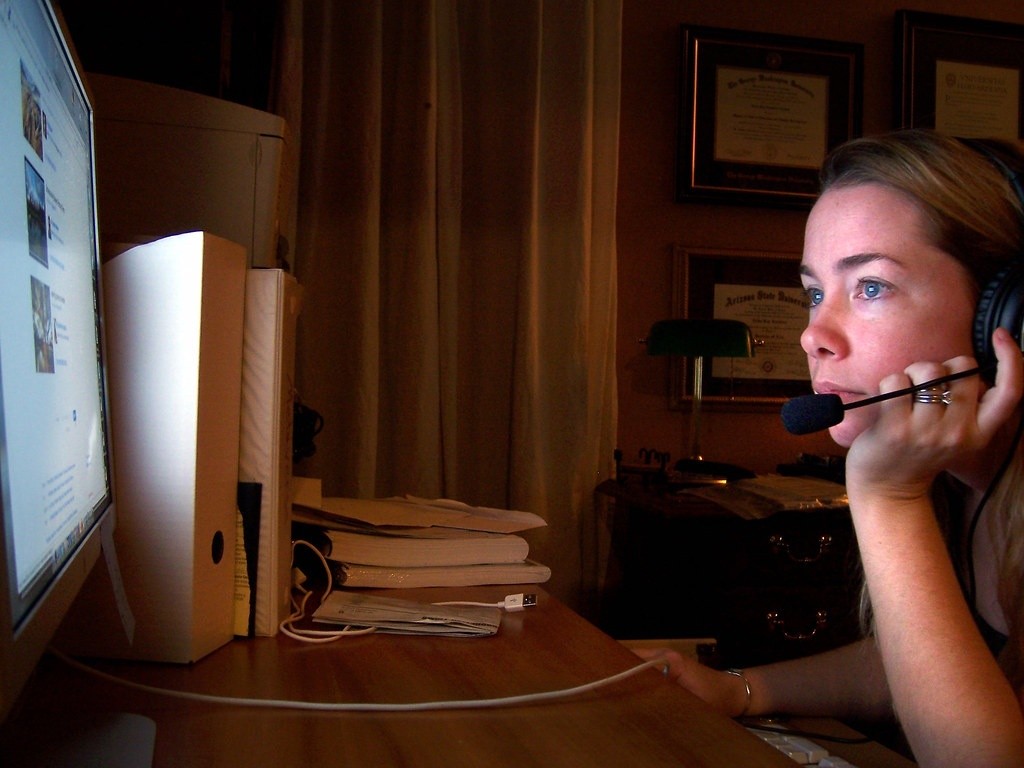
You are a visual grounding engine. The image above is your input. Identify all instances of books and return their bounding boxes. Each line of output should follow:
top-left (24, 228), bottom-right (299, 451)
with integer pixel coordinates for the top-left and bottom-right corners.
top-left (46, 230), bottom-right (550, 665)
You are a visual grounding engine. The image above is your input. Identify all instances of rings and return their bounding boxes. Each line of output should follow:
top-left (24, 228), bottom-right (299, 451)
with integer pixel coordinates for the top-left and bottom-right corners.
top-left (914, 389), bottom-right (951, 405)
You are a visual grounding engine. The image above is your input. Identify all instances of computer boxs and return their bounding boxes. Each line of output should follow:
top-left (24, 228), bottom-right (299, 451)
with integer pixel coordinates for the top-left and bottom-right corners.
top-left (83, 64), bottom-right (298, 277)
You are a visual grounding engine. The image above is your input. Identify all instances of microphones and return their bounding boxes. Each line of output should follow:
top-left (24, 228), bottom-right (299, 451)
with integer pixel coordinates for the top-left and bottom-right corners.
top-left (780, 362), bottom-right (998, 436)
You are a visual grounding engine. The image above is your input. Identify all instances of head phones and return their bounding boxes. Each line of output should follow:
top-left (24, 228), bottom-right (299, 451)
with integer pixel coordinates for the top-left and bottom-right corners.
top-left (950, 136), bottom-right (1024, 390)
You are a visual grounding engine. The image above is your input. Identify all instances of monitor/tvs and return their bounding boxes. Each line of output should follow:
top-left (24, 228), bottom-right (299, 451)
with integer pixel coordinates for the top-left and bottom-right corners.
top-left (0, 0), bottom-right (157, 768)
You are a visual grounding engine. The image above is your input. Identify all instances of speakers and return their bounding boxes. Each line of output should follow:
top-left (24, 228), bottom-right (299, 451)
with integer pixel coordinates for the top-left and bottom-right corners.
top-left (101, 232), bottom-right (247, 665)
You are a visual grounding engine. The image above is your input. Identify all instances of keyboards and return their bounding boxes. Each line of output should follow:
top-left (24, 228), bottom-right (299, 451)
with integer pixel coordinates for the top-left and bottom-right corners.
top-left (742, 724), bottom-right (858, 768)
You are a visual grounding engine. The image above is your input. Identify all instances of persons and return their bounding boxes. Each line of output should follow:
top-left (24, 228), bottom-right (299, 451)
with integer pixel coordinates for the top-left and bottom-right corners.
top-left (628, 132), bottom-right (1024, 768)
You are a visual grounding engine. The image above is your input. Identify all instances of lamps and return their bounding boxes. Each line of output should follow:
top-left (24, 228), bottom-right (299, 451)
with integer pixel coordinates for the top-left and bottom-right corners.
top-left (637, 317), bottom-right (766, 487)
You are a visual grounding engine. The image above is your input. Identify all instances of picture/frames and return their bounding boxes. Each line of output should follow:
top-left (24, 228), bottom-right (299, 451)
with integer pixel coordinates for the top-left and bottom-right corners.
top-left (672, 24), bottom-right (863, 212)
top-left (670, 243), bottom-right (815, 411)
top-left (891, 7), bottom-right (1024, 140)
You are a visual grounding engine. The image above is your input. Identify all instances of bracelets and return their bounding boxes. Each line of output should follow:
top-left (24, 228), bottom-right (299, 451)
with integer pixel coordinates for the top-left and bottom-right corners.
top-left (726, 670), bottom-right (750, 720)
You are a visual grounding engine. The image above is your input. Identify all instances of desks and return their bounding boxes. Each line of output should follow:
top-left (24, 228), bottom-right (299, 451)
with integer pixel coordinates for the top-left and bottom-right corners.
top-left (0, 586), bottom-right (918, 768)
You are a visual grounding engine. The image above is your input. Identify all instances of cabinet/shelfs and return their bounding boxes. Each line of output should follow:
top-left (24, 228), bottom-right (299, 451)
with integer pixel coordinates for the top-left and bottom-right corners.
top-left (593, 478), bottom-right (868, 671)
